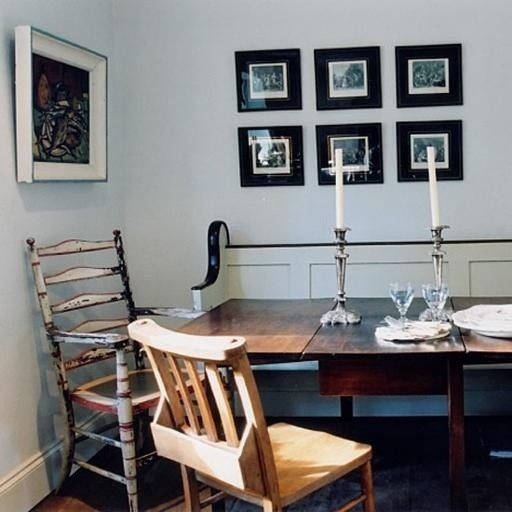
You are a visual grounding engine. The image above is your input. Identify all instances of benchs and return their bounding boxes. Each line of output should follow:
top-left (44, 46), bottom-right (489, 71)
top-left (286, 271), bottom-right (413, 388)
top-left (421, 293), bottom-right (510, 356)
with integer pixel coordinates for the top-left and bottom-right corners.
top-left (191, 220), bottom-right (512, 417)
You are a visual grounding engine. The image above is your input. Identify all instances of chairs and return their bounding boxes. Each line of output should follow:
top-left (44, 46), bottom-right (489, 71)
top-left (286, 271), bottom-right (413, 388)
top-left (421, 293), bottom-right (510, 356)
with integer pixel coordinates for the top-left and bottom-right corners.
top-left (28, 231), bottom-right (225, 512)
top-left (129, 320), bottom-right (375, 512)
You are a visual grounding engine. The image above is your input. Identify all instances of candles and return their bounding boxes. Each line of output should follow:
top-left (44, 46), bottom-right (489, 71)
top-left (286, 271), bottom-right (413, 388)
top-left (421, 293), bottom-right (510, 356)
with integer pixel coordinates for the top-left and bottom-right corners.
top-left (426, 147), bottom-right (440, 228)
top-left (334, 149), bottom-right (346, 228)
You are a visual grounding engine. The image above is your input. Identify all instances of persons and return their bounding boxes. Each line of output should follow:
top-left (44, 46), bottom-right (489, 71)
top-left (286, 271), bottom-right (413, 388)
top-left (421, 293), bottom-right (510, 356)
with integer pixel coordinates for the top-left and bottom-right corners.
top-left (253, 71), bottom-right (283, 92)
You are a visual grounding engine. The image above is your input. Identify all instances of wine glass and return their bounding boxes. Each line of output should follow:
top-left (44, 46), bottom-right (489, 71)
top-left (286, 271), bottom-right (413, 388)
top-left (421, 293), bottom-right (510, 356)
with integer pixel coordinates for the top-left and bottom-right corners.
top-left (388, 281), bottom-right (415, 330)
top-left (421, 283), bottom-right (445, 329)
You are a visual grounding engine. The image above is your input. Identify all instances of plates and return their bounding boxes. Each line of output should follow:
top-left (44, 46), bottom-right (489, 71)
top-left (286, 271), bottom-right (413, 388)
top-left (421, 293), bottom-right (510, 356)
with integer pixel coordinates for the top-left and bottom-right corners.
top-left (451, 303), bottom-right (512, 340)
top-left (374, 321), bottom-right (450, 341)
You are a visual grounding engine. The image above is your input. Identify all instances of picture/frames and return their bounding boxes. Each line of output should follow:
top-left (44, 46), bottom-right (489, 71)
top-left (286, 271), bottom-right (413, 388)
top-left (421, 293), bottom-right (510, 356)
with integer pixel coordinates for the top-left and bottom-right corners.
top-left (13, 26), bottom-right (108, 185)
top-left (235, 42), bottom-right (463, 188)
